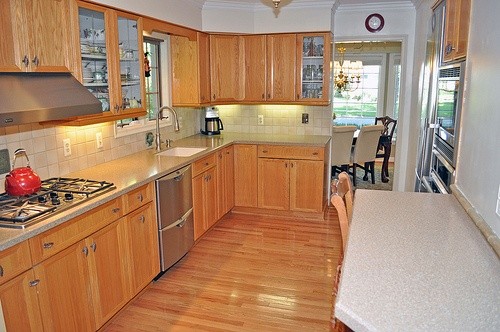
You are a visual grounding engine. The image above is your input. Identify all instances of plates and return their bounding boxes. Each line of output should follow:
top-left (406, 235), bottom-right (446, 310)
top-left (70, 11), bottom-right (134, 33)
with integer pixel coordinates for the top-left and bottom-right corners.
top-left (83, 78), bottom-right (94, 83)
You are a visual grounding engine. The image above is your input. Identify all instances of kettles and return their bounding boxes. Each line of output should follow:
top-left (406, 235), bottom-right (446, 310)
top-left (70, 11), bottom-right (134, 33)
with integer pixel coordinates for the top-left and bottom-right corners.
top-left (5, 149), bottom-right (42, 196)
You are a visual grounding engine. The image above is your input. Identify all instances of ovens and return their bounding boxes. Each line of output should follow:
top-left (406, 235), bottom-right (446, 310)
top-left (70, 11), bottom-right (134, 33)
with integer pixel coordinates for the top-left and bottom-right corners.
top-left (419, 133), bottom-right (455, 193)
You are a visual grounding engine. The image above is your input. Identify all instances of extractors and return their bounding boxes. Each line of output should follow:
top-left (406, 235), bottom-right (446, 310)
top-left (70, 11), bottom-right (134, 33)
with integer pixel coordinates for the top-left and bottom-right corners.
top-left (0, 74), bottom-right (102, 127)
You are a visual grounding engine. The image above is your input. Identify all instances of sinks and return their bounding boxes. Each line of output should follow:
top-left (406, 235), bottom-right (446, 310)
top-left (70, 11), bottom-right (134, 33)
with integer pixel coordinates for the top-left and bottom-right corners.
top-left (153, 146), bottom-right (208, 157)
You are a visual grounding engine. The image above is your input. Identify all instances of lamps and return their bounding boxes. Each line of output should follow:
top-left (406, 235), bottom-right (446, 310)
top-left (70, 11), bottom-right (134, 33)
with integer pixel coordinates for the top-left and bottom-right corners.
top-left (334, 43), bottom-right (360, 93)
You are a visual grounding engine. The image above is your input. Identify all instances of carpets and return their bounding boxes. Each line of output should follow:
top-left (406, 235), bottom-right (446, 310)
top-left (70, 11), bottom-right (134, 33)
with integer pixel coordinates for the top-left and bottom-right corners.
top-left (334, 167), bottom-right (393, 191)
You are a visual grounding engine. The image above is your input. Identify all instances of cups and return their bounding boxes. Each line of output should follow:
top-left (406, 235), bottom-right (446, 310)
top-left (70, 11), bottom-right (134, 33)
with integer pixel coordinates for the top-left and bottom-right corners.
top-left (303, 65), bottom-right (323, 81)
top-left (124, 98), bottom-right (130, 108)
top-left (303, 42), bottom-right (324, 57)
top-left (125, 49), bottom-right (133, 59)
top-left (118, 45), bottom-right (124, 59)
top-left (303, 86), bottom-right (322, 99)
top-left (93, 72), bottom-right (103, 83)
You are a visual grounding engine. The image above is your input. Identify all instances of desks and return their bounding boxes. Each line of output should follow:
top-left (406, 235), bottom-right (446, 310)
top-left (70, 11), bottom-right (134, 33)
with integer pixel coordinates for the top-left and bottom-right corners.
top-left (334, 188), bottom-right (500, 332)
top-left (352, 130), bottom-right (391, 144)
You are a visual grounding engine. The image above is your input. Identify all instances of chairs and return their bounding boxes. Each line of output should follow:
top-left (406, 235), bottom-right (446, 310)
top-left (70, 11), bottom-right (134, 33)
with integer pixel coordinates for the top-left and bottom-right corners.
top-left (351, 125), bottom-right (384, 186)
top-left (332, 126), bottom-right (357, 177)
top-left (330, 171), bottom-right (353, 254)
top-left (375, 116), bottom-right (397, 182)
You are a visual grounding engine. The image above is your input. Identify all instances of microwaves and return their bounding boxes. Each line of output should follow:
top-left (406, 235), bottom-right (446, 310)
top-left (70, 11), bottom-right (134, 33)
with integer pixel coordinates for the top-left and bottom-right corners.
top-left (435, 64), bottom-right (462, 148)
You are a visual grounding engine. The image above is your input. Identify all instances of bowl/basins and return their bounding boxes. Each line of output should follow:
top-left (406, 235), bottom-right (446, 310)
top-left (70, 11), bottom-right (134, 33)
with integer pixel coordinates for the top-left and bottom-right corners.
top-left (82, 69), bottom-right (92, 78)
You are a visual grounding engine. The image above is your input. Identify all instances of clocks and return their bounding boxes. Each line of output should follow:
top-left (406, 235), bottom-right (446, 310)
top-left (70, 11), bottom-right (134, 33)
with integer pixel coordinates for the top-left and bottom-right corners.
top-left (365, 13), bottom-right (384, 33)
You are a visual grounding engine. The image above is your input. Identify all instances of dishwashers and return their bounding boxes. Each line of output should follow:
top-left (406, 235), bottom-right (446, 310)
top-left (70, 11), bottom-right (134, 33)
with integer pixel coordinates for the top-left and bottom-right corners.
top-left (154, 163), bottom-right (194, 272)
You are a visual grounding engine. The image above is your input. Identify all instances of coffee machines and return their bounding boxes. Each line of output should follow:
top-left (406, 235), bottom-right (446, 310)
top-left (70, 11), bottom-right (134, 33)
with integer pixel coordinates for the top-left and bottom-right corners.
top-left (205, 108), bottom-right (224, 135)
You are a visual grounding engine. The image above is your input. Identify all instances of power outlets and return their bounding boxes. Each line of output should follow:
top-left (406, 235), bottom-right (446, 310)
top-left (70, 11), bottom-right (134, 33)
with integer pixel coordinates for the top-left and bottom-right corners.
top-left (258, 115), bottom-right (263, 125)
top-left (63, 139), bottom-right (71, 157)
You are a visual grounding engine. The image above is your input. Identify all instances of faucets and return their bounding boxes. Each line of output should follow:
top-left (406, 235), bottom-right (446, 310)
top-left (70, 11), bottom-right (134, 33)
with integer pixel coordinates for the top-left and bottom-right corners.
top-left (155, 104), bottom-right (180, 152)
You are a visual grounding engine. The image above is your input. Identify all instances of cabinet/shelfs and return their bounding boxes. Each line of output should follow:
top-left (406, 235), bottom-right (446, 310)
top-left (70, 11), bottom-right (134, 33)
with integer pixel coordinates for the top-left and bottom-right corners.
top-left (210, 32), bottom-right (245, 105)
top-left (257, 142), bottom-right (330, 222)
top-left (171, 24), bottom-right (210, 107)
top-left (0, 181), bottom-right (161, 332)
top-left (233, 143), bottom-right (257, 215)
top-left (245, 32), bottom-right (297, 104)
top-left (216, 144), bottom-right (235, 224)
top-left (0, 0), bottom-right (74, 72)
top-left (297, 31), bottom-right (334, 106)
top-left (442, 0), bottom-right (471, 62)
top-left (191, 152), bottom-right (218, 245)
top-left (39, 0), bottom-right (146, 127)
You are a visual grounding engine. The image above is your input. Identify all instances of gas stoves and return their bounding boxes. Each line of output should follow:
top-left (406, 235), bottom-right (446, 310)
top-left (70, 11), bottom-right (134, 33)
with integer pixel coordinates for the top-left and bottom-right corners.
top-left (0, 178), bottom-right (116, 230)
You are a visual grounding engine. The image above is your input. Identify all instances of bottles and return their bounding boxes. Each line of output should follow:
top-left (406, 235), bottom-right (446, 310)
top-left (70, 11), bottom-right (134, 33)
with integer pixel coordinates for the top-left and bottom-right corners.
top-left (98, 98), bottom-right (109, 112)
